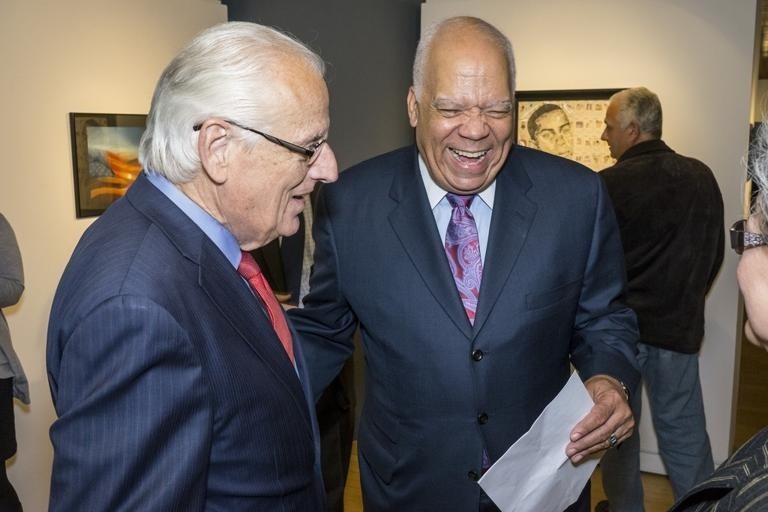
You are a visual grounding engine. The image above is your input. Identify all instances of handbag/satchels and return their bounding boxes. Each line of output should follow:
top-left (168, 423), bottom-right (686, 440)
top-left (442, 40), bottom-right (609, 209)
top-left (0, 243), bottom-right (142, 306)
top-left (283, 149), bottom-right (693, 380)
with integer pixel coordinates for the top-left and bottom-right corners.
top-left (620, 381), bottom-right (629, 396)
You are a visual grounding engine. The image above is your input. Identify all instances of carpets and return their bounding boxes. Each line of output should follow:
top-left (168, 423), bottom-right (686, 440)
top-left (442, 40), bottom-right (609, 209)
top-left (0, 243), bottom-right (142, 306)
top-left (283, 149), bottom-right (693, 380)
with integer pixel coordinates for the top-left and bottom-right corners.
top-left (237, 251), bottom-right (294, 375)
top-left (444, 193), bottom-right (483, 328)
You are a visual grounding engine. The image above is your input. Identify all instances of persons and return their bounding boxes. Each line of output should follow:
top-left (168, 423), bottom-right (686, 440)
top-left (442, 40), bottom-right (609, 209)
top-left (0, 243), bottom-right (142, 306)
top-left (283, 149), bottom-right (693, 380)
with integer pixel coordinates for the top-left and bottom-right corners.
top-left (0, 213), bottom-right (31, 512)
top-left (594, 86), bottom-right (724, 512)
top-left (46, 20), bottom-right (339, 512)
top-left (659, 113), bottom-right (768, 512)
top-left (286, 16), bottom-right (648, 512)
top-left (528, 104), bottom-right (574, 159)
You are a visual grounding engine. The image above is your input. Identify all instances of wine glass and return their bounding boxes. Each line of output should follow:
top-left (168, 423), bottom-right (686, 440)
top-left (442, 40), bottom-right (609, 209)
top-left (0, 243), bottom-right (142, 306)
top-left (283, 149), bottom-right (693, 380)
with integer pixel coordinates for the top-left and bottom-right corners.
top-left (608, 433), bottom-right (617, 447)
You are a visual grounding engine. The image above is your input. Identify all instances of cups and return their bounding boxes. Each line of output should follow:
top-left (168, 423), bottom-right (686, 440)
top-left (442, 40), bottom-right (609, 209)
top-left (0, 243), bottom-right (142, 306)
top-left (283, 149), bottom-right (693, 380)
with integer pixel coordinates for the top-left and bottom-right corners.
top-left (729, 219), bottom-right (768, 255)
top-left (193, 119), bottom-right (327, 165)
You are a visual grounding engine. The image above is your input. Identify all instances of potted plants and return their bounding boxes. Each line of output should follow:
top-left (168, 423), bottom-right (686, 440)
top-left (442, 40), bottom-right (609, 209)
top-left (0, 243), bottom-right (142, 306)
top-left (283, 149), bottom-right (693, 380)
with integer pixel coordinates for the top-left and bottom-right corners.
top-left (510, 88), bottom-right (630, 174)
top-left (69, 112), bottom-right (148, 219)
